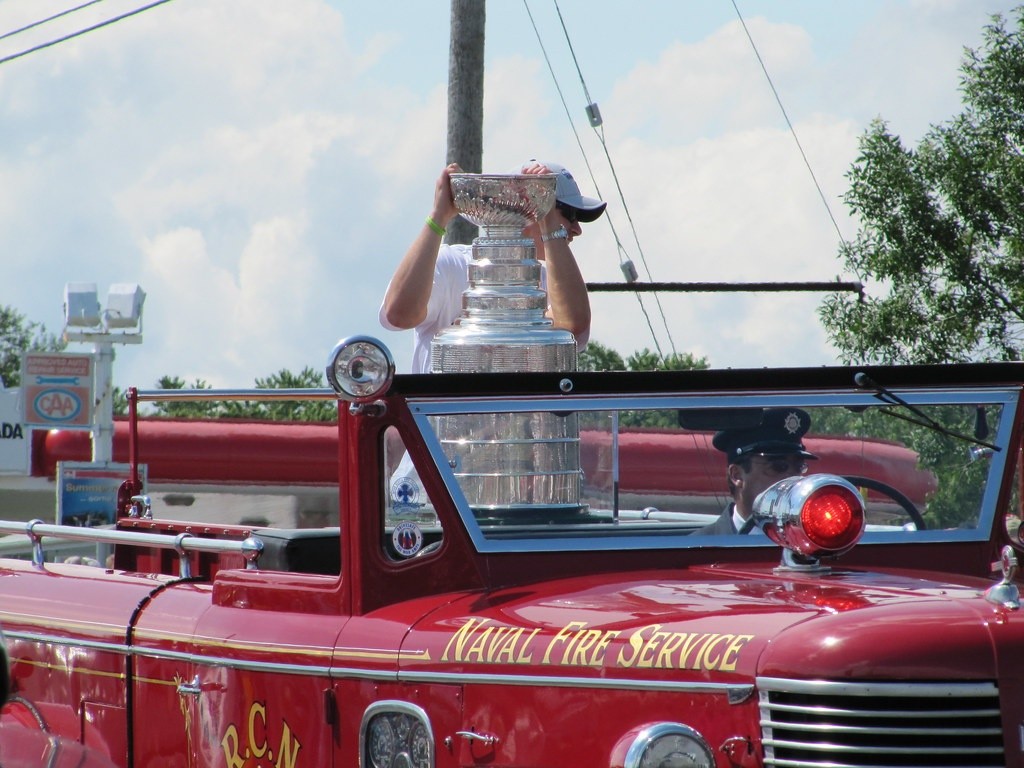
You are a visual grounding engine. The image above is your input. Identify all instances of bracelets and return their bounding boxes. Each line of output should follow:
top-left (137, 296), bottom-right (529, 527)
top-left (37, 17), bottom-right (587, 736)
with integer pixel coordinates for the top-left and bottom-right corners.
top-left (425, 216), bottom-right (446, 236)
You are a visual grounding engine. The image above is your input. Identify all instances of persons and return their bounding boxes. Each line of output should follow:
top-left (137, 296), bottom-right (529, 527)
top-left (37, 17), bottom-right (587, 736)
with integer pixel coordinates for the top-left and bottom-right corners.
top-left (688, 408), bottom-right (819, 535)
top-left (379, 158), bottom-right (607, 515)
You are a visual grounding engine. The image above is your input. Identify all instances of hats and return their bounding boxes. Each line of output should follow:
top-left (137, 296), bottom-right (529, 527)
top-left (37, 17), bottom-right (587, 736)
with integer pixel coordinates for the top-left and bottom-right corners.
top-left (509, 158), bottom-right (608, 222)
top-left (713, 407), bottom-right (819, 464)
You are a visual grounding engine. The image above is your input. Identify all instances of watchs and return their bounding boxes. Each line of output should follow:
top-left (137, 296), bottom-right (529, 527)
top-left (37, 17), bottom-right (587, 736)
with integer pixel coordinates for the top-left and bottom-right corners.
top-left (540, 223), bottom-right (568, 242)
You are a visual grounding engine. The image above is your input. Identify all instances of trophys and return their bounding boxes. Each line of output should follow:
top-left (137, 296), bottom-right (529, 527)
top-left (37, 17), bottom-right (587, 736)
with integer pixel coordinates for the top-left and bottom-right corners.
top-left (423, 172), bottom-right (578, 508)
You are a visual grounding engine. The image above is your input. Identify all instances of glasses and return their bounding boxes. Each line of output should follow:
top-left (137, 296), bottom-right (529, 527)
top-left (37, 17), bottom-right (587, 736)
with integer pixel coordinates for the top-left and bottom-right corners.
top-left (555, 201), bottom-right (576, 222)
top-left (746, 458), bottom-right (810, 479)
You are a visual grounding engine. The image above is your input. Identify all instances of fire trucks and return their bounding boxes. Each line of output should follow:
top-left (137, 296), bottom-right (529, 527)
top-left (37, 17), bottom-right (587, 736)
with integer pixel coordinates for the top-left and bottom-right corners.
top-left (0, 167), bottom-right (1024, 768)
